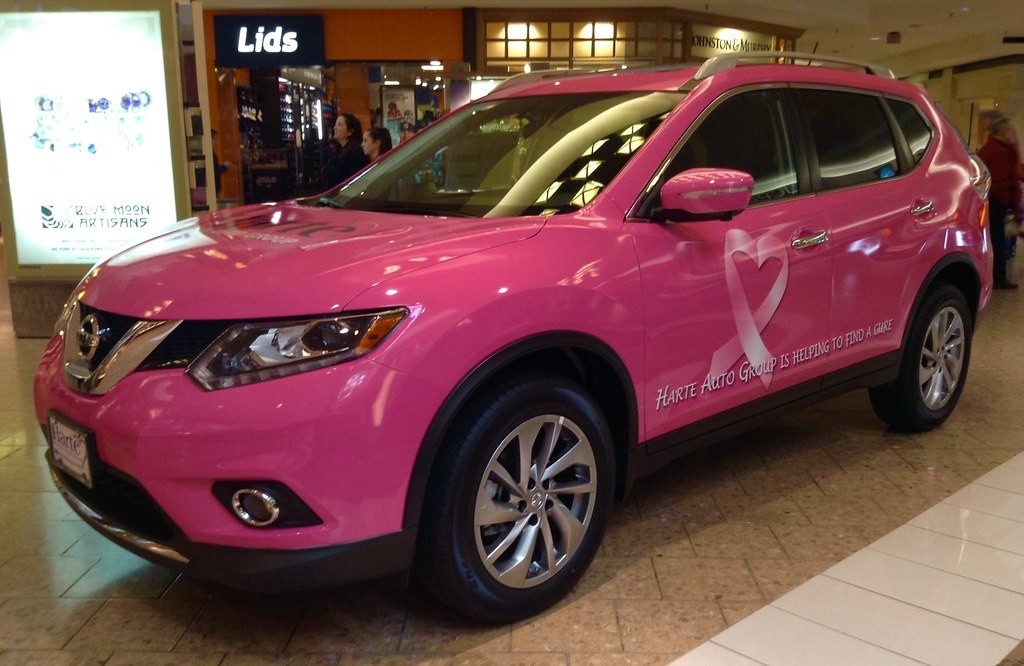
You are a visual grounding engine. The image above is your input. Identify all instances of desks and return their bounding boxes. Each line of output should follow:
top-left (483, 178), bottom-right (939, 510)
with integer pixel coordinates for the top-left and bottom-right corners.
top-left (242, 146), bottom-right (302, 204)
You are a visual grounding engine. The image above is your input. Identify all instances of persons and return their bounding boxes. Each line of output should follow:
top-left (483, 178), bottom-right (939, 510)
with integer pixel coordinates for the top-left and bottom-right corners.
top-left (321, 113), bottom-right (438, 191)
top-left (976, 111), bottom-right (1024, 290)
top-left (195, 128), bottom-right (230, 198)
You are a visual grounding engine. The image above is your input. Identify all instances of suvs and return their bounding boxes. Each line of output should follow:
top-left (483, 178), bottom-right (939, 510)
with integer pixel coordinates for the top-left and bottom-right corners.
top-left (29, 49), bottom-right (997, 626)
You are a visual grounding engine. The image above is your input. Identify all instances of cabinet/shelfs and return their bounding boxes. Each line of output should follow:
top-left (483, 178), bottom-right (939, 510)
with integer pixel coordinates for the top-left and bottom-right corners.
top-left (249, 65), bottom-right (337, 202)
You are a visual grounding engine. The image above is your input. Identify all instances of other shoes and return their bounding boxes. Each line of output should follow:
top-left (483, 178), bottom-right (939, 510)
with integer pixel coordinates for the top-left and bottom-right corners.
top-left (992, 281), bottom-right (1018, 290)
